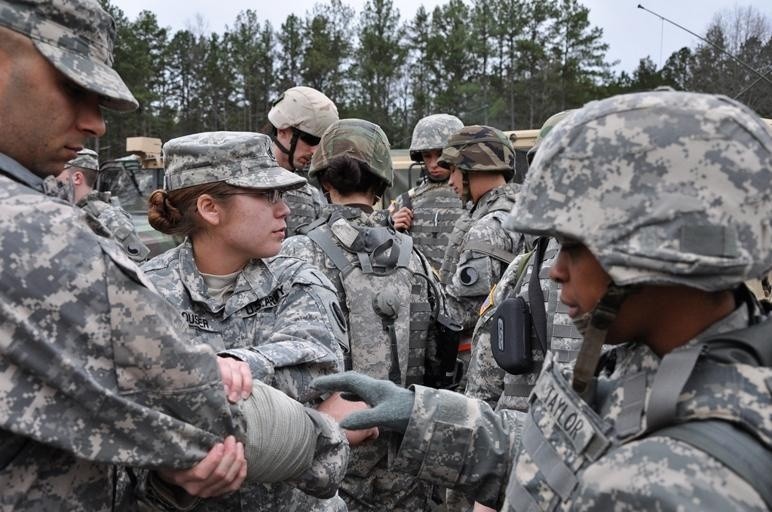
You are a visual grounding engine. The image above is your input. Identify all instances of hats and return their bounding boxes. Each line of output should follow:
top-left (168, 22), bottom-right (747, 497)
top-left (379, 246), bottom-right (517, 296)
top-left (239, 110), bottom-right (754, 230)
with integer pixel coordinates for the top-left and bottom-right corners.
top-left (161, 129), bottom-right (309, 192)
top-left (63, 147), bottom-right (100, 172)
top-left (0, 0), bottom-right (140, 114)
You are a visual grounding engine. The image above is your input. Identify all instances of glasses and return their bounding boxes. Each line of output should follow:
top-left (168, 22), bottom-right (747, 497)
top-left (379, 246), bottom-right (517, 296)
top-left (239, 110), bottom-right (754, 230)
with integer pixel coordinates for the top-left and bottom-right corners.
top-left (213, 187), bottom-right (287, 203)
top-left (290, 126), bottom-right (322, 146)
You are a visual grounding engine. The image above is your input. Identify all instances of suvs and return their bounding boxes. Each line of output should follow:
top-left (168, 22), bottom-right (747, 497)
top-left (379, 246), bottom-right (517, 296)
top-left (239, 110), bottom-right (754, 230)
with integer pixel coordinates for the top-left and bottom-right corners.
top-left (88, 154), bottom-right (185, 262)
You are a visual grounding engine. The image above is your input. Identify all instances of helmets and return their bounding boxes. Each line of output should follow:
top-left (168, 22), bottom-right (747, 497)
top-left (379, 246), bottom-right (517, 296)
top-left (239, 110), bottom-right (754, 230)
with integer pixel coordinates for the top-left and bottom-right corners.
top-left (267, 86), bottom-right (339, 139)
top-left (409, 113), bottom-right (465, 162)
top-left (306, 117), bottom-right (395, 189)
top-left (527, 106), bottom-right (581, 170)
top-left (437, 124), bottom-right (517, 184)
top-left (500, 89), bottom-right (772, 297)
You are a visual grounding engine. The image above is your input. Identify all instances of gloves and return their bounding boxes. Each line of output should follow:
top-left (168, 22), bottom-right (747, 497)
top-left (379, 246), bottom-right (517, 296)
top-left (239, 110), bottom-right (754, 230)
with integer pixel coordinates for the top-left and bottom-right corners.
top-left (308, 369), bottom-right (416, 436)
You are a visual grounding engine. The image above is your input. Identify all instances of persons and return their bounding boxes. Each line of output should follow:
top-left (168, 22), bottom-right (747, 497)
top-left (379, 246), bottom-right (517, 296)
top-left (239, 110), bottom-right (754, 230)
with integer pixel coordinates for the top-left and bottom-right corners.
top-left (259, 87), bottom-right (772, 511)
top-left (0, 0), bottom-right (350, 511)
